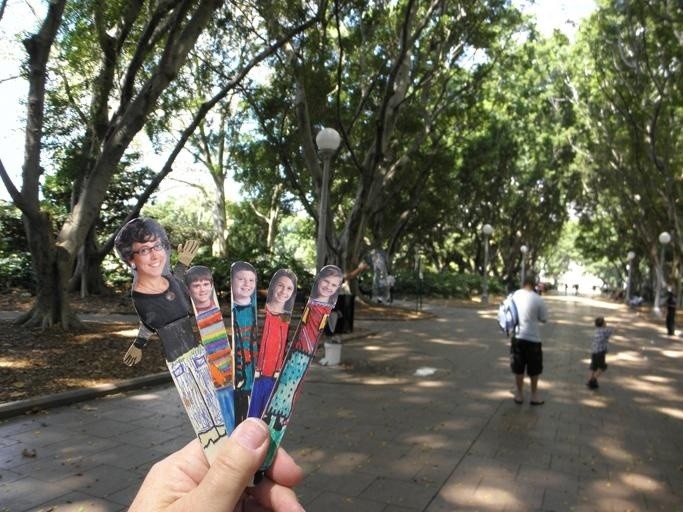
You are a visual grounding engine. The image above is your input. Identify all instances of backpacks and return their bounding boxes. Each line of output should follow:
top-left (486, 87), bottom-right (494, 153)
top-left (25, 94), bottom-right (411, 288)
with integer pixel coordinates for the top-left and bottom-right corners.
top-left (496, 289), bottom-right (520, 340)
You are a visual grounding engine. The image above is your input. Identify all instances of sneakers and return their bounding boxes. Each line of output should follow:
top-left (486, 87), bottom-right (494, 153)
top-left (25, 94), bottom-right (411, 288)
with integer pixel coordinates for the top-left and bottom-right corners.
top-left (585, 376), bottom-right (599, 389)
top-left (514, 396), bottom-right (545, 405)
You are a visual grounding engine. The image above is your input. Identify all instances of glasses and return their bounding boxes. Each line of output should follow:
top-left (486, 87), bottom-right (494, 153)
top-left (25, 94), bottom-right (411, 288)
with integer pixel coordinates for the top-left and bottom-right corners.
top-left (132, 241), bottom-right (166, 257)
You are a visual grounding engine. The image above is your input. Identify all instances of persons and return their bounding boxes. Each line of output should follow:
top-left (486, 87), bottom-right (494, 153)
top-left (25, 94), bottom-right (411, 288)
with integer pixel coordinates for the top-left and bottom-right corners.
top-left (183, 265), bottom-right (234, 438)
top-left (584, 315), bottom-right (616, 389)
top-left (261, 264), bottom-right (345, 432)
top-left (503, 273), bottom-right (548, 407)
top-left (124, 416), bottom-right (304, 511)
top-left (663, 283), bottom-right (677, 336)
top-left (246, 266), bottom-right (297, 418)
top-left (227, 258), bottom-right (257, 426)
top-left (111, 216), bottom-right (227, 451)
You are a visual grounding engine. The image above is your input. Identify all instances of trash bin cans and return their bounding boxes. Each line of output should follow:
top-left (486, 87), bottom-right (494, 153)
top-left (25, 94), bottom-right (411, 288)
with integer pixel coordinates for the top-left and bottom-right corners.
top-left (325, 294), bottom-right (354, 334)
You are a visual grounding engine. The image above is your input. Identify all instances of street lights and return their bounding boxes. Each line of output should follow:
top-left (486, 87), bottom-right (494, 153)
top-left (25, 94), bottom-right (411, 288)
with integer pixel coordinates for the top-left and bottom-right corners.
top-left (520, 245), bottom-right (528, 287)
top-left (482, 224), bottom-right (493, 306)
top-left (315, 127), bottom-right (341, 276)
top-left (654, 230), bottom-right (672, 311)
top-left (626, 250), bottom-right (636, 305)
